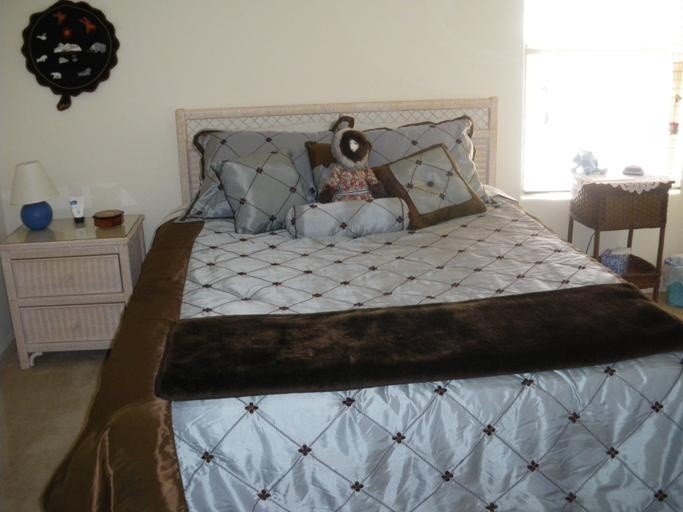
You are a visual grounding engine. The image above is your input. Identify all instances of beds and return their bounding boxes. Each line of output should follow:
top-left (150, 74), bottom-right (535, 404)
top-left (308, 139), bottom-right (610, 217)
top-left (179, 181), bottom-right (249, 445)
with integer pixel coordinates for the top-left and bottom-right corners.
top-left (91, 94), bottom-right (683, 511)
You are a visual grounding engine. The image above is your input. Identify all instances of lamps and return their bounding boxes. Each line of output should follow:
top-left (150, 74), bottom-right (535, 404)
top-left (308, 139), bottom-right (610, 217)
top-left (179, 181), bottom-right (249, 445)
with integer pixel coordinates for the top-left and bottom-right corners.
top-left (8, 160), bottom-right (59, 230)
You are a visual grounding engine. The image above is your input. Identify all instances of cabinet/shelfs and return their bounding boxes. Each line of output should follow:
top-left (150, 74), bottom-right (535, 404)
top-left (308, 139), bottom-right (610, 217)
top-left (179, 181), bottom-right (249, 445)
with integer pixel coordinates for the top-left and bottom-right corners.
top-left (568, 175), bottom-right (673, 302)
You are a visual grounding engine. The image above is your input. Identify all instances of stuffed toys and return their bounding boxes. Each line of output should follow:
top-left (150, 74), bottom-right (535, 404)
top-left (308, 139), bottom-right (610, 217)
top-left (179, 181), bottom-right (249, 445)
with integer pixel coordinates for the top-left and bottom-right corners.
top-left (319, 115), bottom-right (383, 203)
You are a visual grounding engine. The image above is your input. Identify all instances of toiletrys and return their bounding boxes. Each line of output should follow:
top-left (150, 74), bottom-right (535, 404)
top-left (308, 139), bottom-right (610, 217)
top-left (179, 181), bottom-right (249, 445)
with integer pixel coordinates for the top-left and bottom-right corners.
top-left (69, 196), bottom-right (86, 225)
top-left (68, 197), bottom-right (85, 223)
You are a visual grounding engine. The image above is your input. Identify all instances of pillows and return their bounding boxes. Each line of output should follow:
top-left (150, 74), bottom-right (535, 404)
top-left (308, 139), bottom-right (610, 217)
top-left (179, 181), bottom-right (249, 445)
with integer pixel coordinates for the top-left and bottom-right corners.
top-left (209, 149), bottom-right (316, 233)
top-left (362, 117), bottom-right (498, 205)
top-left (371, 142), bottom-right (487, 229)
top-left (304, 141), bottom-right (387, 198)
top-left (175, 130), bottom-right (335, 224)
top-left (286, 197), bottom-right (411, 234)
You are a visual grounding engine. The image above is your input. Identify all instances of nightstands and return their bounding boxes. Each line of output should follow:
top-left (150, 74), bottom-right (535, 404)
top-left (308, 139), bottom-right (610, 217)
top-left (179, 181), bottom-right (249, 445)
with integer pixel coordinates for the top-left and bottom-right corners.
top-left (0, 213), bottom-right (145, 369)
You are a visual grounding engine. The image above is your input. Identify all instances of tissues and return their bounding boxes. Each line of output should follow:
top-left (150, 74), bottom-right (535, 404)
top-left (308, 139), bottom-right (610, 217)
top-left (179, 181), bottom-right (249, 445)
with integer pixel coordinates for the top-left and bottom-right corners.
top-left (600, 247), bottom-right (632, 276)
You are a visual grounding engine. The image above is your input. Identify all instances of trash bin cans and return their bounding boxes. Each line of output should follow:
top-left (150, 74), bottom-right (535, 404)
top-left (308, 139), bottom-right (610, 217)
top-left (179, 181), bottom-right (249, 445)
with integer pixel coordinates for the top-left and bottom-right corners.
top-left (664, 255), bottom-right (683, 308)
